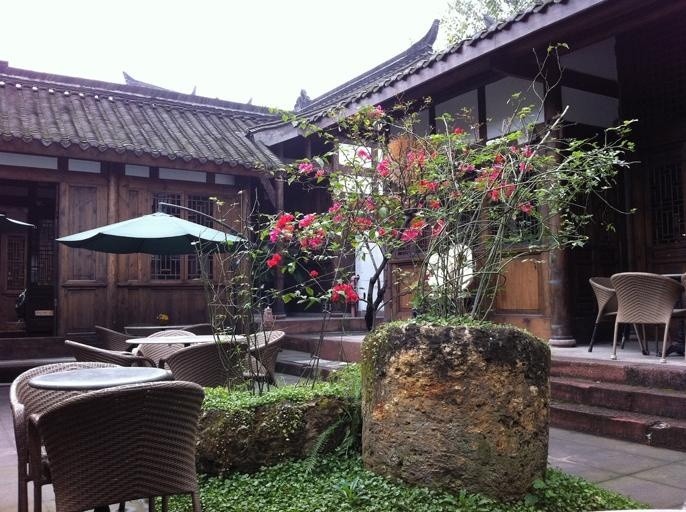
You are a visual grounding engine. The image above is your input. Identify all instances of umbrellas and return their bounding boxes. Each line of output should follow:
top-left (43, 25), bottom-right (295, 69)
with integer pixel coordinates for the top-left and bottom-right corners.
top-left (52, 198), bottom-right (257, 334)
top-left (0, 212), bottom-right (37, 235)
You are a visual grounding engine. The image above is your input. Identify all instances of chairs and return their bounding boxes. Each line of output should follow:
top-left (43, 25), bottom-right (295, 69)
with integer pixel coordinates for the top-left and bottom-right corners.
top-left (27, 380), bottom-right (204, 511)
top-left (587, 270), bottom-right (686, 364)
top-left (9, 362), bottom-right (126, 510)
top-left (65, 322), bottom-right (286, 388)
top-left (426, 244), bottom-right (475, 306)
